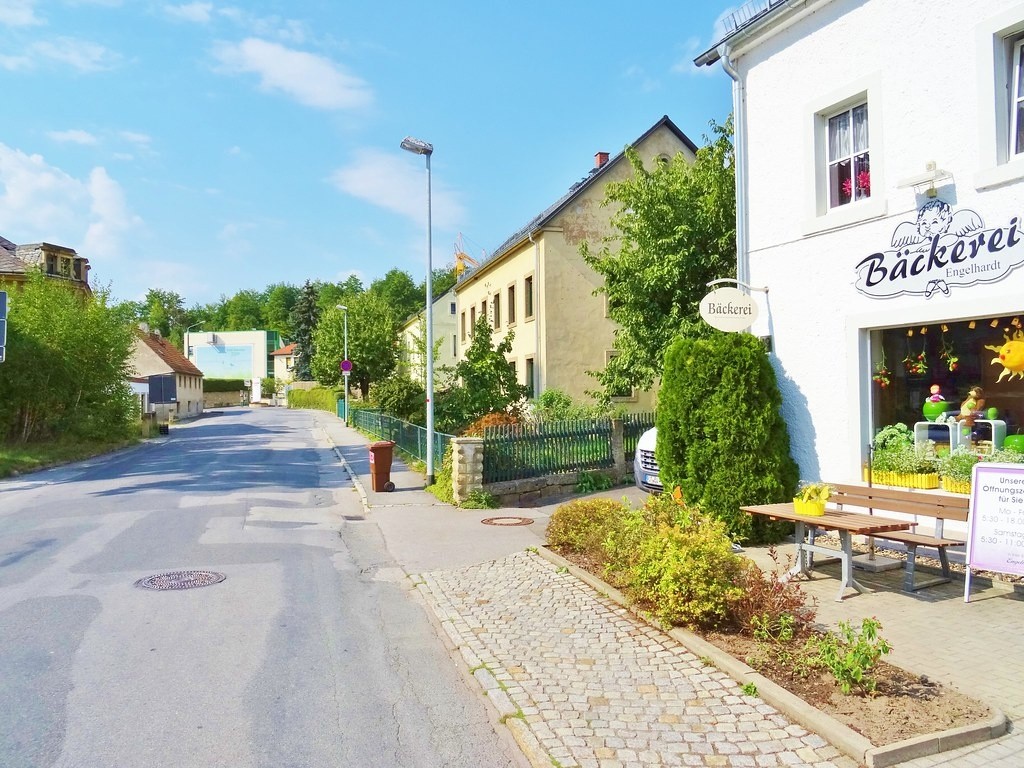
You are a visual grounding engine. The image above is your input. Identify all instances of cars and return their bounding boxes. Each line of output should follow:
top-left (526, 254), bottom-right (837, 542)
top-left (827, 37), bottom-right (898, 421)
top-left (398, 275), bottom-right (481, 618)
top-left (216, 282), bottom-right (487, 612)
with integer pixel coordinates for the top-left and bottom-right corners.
top-left (634, 426), bottom-right (663, 496)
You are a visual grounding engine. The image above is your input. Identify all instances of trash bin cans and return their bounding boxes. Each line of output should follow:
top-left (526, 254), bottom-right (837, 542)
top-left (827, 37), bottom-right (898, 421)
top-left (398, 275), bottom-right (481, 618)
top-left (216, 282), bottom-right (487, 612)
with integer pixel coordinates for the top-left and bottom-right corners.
top-left (366, 440), bottom-right (397, 492)
top-left (141, 410), bottom-right (157, 438)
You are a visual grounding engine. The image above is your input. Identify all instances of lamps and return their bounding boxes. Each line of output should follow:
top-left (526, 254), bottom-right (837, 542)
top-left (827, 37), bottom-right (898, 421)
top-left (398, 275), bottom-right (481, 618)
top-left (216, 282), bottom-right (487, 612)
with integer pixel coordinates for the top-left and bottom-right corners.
top-left (941, 324), bottom-right (948, 332)
top-left (969, 321), bottom-right (976, 329)
top-left (920, 326), bottom-right (928, 334)
top-left (990, 318), bottom-right (999, 328)
top-left (1011, 315), bottom-right (1019, 326)
top-left (907, 327), bottom-right (913, 337)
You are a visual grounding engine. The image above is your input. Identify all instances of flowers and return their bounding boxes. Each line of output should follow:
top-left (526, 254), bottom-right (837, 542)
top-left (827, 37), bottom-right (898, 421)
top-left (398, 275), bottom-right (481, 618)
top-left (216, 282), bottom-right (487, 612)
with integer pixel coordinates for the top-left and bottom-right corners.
top-left (857, 170), bottom-right (870, 187)
top-left (842, 178), bottom-right (851, 197)
top-left (872, 330), bottom-right (891, 390)
top-left (917, 327), bottom-right (928, 374)
top-left (938, 324), bottom-right (958, 371)
top-left (796, 482), bottom-right (830, 503)
top-left (902, 328), bottom-right (918, 373)
top-left (940, 445), bottom-right (1024, 485)
top-left (873, 424), bottom-right (939, 475)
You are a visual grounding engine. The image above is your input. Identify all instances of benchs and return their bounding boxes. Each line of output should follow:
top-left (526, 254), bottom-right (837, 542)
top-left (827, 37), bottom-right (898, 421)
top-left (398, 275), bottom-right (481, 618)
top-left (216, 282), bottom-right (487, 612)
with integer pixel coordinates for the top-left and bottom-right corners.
top-left (819, 482), bottom-right (970, 592)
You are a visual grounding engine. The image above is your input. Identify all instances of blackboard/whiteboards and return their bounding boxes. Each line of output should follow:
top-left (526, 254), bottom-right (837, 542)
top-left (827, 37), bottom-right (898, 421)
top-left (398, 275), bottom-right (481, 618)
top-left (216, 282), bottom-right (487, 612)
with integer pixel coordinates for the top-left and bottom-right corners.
top-left (963, 462), bottom-right (1024, 577)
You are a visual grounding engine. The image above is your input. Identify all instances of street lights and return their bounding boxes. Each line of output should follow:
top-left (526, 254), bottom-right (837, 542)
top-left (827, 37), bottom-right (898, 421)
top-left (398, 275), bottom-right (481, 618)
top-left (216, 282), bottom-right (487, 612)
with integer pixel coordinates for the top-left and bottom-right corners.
top-left (400, 136), bottom-right (435, 487)
top-left (187, 320), bottom-right (207, 361)
top-left (337, 303), bottom-right (349, 427)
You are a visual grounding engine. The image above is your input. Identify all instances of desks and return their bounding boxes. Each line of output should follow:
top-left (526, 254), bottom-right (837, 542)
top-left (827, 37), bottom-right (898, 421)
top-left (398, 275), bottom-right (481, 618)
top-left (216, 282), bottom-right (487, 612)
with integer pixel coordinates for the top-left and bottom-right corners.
top-left (740, 503), bottom-right (919, 602)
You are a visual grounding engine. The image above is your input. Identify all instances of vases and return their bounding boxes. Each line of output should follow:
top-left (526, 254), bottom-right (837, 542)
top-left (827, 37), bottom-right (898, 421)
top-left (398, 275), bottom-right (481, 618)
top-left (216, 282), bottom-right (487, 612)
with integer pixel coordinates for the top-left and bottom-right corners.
top-left (942, 475), bottom-right (972, 494)
top-left (793, 498), bottom-right (825, 516)
top-left (850, 187), bottom-right (866, 203)
top-left (864, 462), bottom-right (939, 490)
